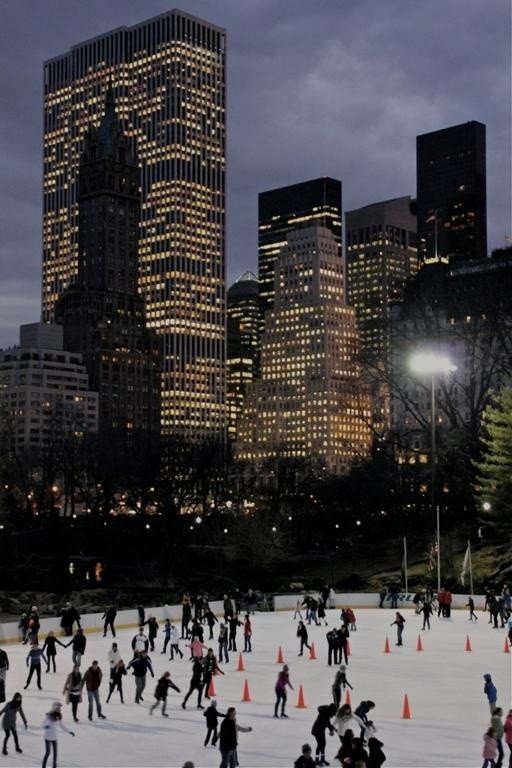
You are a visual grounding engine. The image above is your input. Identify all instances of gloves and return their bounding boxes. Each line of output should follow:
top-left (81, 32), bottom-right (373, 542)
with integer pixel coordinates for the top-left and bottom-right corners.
top-left (44, 724), bottom-right (51, 729)
top-left (69, 731), bottom-right (75, 737)
top-left (24, 724), bottom-right (28, 730)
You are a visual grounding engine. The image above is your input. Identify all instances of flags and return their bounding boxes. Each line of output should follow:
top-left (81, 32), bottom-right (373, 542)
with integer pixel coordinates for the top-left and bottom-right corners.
top-left (461, 549), bottom-right (468, 586)
top-left (427, 542), bottom-right (437, 574)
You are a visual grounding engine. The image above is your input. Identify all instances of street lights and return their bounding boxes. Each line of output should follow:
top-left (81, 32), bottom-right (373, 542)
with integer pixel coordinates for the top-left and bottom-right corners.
top-left (483, 502), bottom-right (492, 542)
top-left (411, 350), bottom-right (456, 590)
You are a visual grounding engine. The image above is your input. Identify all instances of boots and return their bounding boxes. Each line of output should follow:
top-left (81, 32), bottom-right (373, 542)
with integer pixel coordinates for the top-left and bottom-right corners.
top-left (2, 745), bottom-right (8, 755)
top-left (16, 745), bottom-right (22, 753)
top-left (320, 755), bottom-right (330, 765)
top-left (314, 756), bottom-right (323, 766)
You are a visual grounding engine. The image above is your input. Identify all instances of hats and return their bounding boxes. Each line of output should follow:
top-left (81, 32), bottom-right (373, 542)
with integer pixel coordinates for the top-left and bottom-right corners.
top-left (340, 665), bottom-right (346, 670)
top-left (211, 700), bottom-right (217, 705)
top-left (301, 744), bottom-right (311, 753)
top-left (51, 701), bottom-right (62, 711)
top-left (282, 665), bottom-right (288, 671)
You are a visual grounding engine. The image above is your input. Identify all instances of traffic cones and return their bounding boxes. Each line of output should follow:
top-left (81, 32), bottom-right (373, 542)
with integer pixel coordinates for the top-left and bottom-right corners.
top-left (308, 642), bottom-right (317, 659)
top-left (294, 684), bottom-right (307, 710)
top-left (343, 690), bottom-right (351, 707)
top-left (345, 640), bottom-right (351, 655)
top-left (503, 637), bottom-right (510, 653)
top-left (213, 658), bottom-right (220, 676)
top-left (400, 694), bottom-right (411, 720)
top-left (464, 635), bottom-right (472, 652)
top-left (382, 636), bottom-right (391, 653)
top-left (237, 652), bottom-right (246, 672)
top-left (276, 645), bottom-right (284, 665)
top-left (241, 678), bottom-right (252, 703)
top-left (416, 634), bottom-right (422, 652)
top-left (207, 676), bottom-right (217, 698)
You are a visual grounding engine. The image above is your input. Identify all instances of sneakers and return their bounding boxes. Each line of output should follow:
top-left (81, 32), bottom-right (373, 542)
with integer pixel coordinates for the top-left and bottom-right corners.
top-left (327, 660), bottom-right (348, 666)
top-left (98, 713), bottom-right (105, 718)
top-left (53, 667), bottom-right (56, 672)
top-left (181, 701), bottom-right (185, 709)
top-left (105, 696), bottom-right (169, 719)
top-left (204, 741), bottom-right (207, 746)
top-left (150, 646), bottom-right (183, 661)
top-left (24, 684), bottom-right (29, 689)
top-left (102, 633), bottom-right (106, 638)
top-left (38, 685), bottom-right (42, 689)
top-left (298, 653), bottom-right (303, 656)
top-left (112, 633), bottom-right (116, 637)
top-left (218, 647), bottom-right (252, 665)
top-left (415, 609), bottom-right (451, 630)
top-left (273, 713), bottom-right (279, 718)
top-left (45, 668), bottom-right (50, 672)
top-left (396, 643), bottom-right (402, 646)
top-left (294, 616), bottom-right (329, 627)
top-left (88, 714), bottom-right (92, 720)
top-left (469, 614), bottom-right (508, 628)
top-left (179, 620), bottom-right (214, 642)
top-left (197, 703), bottom-right (204, 708)
top-left (281, 712), bottom-right (288, 717)
top-left (211, 743), bottom-right (216, 746)
